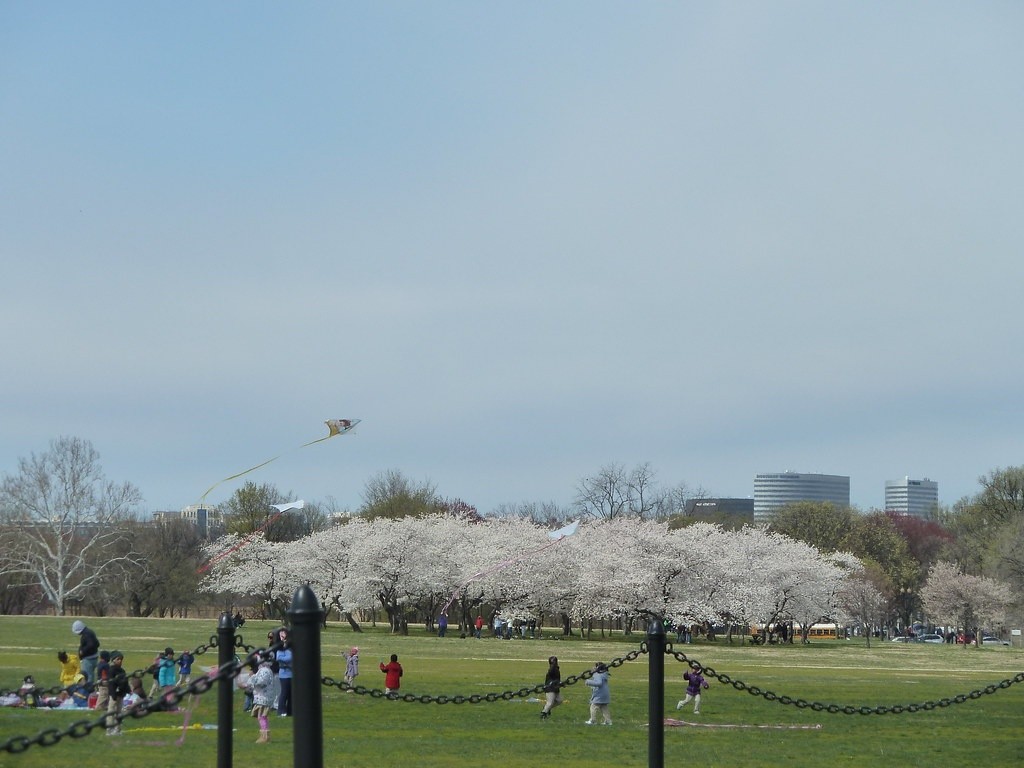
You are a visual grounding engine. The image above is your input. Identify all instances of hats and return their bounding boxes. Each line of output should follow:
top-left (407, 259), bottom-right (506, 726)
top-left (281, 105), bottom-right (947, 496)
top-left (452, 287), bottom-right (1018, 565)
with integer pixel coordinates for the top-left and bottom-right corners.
top-left (72, 620), bottom-right (86, 634)
top-left (164, 647), bottom-right (175, 656)
top-left (350, 647), bottom-right (359, 655)
top-left (110, 650), bottom-right (123, 661)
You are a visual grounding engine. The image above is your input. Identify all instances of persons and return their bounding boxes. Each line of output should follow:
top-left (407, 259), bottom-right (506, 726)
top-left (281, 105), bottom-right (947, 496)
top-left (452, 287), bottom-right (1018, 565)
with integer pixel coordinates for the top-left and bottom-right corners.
top-left (585, 662), bottom-right (612, 725)
top-left (894, 627), bottom-right (955, 641)
top-left (475, 616), bottom-right (482, 639)
top-left (752, 623), bottom-right (788, 645)
top-left (438, 612), bottom-right (447, 637)
top-left (233, 612), bottom-right (245, 628)
top-left (149, 647), bottom-right (195, 698)
top-left (677, 664), bottom-right (709, 714)
top-left (341, 647), bottom-right (358, 693)
top-left (541, 656), bottom-right (563, 719)
top-left (380, 655), bottom-right (403, 700)
top-left (677, 625), bottom-right (691, 643)
top-left (58, 621), bottom-right (146, 736)
top-left (20, 675), bottom-right (43, 706)
top-left (240, 629), bottom-right (292, 743)
top-left (495, 615), bottom-right (536, 639)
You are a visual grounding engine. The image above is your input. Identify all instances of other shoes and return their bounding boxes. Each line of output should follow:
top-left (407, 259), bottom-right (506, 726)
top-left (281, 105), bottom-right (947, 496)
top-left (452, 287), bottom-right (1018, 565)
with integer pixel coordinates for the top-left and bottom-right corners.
top-left (584, 720), bottom-right (597, 725)
top-left (694, 709), bottom-right (700, 714)
top-left (600, 722), bottom-right (612, 726)
top-left (541, 711), bottom-right (551, 719)
top-left (106, 728), bottom-right (123, 735)
top-left (677, 702), bottom-right (683, 710)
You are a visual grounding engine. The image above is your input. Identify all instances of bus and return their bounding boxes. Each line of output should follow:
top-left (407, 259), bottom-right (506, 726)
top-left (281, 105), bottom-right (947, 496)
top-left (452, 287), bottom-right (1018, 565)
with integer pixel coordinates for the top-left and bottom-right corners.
top-left (751, 623), bottom-right (843, 639)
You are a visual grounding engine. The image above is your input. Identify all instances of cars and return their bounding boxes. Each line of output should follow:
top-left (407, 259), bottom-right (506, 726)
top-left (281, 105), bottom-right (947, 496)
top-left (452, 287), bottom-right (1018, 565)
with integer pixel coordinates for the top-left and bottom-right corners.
top-left (917, 634), bottom-right (947, 644)
top-left (891, 636), bottom-right (913, 643)
top-left (970, 637), bottom-right (1009, 646)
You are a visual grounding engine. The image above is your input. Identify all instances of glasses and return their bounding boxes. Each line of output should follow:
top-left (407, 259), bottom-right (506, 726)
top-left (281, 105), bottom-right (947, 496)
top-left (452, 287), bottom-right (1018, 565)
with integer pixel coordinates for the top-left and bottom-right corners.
top-left (268, 636), bottom-right (272, 639)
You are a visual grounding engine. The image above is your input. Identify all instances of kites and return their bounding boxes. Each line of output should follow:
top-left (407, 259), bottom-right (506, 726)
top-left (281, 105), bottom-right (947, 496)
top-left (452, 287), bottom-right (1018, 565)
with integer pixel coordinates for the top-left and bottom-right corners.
top-left (441, 519), bottom-right (580, 614)
top-left (198, 418), bottom-right (362, 501)
top-left (195, 500), bottom-right (304, 575)
top-left (111, 664), bottom-right (217, 746)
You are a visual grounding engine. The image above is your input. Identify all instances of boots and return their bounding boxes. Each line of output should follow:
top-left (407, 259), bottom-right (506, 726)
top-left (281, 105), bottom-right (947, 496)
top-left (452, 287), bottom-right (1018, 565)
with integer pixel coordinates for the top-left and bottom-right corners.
top-left (255, 729), bottom-right (270, 745)
